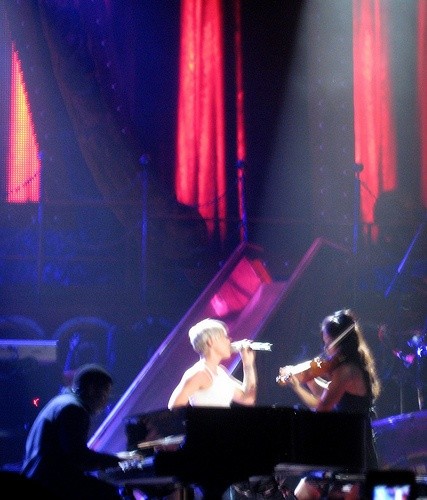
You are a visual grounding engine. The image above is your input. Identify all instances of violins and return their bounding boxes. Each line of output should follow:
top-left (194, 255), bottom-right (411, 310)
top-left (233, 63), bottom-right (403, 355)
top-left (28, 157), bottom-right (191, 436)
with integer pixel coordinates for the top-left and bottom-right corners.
top-left (275, 353), bottom-right (340, 389)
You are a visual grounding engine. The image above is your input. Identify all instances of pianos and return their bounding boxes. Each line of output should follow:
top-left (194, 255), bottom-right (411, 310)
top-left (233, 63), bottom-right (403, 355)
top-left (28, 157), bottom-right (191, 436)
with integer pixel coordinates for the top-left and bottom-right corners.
top-left (103, 404), bottom-right (370, 500)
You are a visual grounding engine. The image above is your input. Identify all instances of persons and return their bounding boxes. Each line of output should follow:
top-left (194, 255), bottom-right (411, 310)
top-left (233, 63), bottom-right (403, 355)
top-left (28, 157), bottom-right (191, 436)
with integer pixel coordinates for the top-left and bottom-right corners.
top-left (19, 364), bottom-right (144, 499)
top-left (166, 317), bottom-right (273, 408)
top-left (275, 307), bottom-right (381, 500)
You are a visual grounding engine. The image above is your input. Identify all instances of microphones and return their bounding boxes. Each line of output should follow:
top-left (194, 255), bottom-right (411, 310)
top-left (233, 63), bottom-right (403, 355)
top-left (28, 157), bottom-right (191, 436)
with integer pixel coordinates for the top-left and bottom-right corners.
top-left (232, 340), bottom-right (274, 352)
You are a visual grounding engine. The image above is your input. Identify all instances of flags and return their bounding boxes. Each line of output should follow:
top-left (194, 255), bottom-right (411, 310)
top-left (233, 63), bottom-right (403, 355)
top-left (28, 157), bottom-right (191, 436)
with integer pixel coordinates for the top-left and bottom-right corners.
top-left (353, 0), bottom-right (398, 225)
top-left (176, 0), bottom-right (228, 221)
top-left (7, 42), bottom-right (41, 202)
top-left (418, 0), bottom-right (427, 209)
